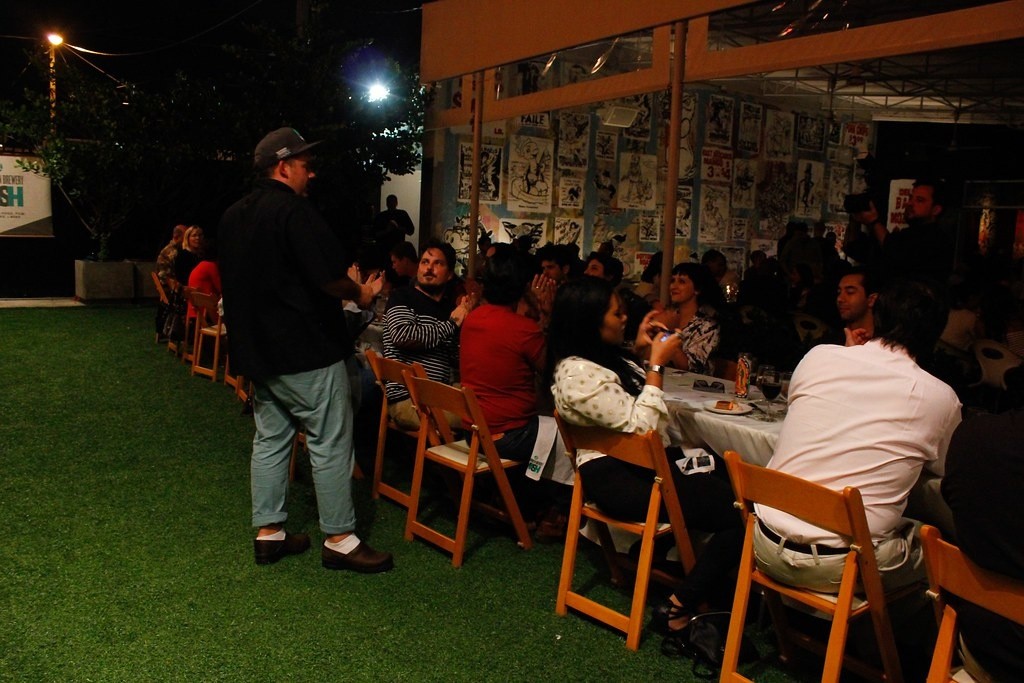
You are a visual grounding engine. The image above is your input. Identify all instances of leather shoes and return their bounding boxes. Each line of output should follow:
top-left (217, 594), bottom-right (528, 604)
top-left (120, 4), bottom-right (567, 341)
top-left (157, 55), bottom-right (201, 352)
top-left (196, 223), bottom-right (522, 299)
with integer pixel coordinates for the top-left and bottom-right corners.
top-left (322, 538), bottom-right (394, 573)
top-left (253, 530), bottom-right (312, 564)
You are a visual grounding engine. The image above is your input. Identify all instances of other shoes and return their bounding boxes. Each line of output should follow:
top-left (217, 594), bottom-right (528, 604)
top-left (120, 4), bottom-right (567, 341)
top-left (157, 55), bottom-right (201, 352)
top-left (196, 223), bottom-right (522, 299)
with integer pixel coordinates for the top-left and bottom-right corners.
top-left (647, 596), bottom-right (692, 637)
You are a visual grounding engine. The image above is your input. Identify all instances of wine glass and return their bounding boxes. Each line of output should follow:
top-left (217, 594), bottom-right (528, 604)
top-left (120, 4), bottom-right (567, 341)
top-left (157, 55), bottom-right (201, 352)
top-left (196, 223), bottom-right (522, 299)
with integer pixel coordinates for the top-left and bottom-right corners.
top-left (758, 370), bottom-right (782, 422)
top-left (756, 366), bottom-right (778, 405)
top-left (779, 371), bottom-right (794, 415)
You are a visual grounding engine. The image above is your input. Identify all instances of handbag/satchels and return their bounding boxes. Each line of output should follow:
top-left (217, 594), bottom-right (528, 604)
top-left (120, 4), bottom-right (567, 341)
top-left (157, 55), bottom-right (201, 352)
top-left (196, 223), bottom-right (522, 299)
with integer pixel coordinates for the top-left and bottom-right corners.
top-left (660, 612), bottom-right (761, 680)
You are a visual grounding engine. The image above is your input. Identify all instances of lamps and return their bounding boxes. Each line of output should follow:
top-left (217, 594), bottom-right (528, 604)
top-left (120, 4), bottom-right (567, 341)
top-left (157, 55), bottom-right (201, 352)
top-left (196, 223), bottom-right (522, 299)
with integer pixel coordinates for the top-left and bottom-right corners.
top-left (595, 100), bottom-right (638, 128)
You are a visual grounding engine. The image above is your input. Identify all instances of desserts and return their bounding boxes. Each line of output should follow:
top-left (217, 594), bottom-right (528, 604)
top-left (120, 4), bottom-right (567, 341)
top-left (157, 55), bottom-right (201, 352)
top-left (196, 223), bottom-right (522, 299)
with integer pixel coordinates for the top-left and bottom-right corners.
top-left (716, 400), bottom-right (733, 409)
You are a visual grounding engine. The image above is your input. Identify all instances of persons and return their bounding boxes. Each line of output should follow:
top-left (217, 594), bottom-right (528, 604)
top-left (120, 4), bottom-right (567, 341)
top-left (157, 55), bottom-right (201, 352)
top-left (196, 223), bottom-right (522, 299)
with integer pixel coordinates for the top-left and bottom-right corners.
top-left (156, 224), bottom-right (221, 324)
top-left (373, 195), bottom-right (414, 255)
top-left (221, 128), bottom-right (392, 574)
top-left (382, 173), bottom-right (1024, 683)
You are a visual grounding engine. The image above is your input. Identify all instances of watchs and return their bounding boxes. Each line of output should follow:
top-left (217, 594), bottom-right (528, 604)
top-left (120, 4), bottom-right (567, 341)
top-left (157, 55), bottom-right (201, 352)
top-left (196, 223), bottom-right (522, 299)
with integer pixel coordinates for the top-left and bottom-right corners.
top-left (648, 365), bottom-right (664, 375)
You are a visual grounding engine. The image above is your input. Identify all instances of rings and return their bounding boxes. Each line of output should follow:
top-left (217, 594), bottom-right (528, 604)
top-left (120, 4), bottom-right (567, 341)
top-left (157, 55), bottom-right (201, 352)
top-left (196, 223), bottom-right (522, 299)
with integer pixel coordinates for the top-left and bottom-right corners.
top-left (649, 322), bottom-right (653, 327)
top-left (536, 286), bottom-right (540, 289)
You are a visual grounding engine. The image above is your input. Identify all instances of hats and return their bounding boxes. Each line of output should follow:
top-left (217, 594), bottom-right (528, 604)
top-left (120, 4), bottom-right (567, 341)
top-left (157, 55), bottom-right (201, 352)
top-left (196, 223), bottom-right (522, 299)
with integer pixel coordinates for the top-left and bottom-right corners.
top-left (255, 127), bottom-right (326, 172)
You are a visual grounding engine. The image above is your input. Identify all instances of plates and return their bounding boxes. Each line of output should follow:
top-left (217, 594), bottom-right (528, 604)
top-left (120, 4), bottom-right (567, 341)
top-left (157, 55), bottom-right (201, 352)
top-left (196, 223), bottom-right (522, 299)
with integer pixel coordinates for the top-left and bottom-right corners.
top-left (703, 399), bottom-right (753, 415)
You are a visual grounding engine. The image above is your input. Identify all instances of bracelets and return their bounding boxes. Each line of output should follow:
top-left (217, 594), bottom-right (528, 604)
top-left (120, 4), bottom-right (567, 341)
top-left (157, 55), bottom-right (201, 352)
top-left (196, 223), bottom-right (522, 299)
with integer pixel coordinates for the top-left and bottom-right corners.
top-left (871, 218), bottom-right (881, 225)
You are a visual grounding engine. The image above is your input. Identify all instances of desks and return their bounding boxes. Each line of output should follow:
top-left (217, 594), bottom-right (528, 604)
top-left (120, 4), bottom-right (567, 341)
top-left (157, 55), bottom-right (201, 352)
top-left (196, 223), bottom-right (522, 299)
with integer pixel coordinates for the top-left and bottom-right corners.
top-left (363, 323), bottom-right (954, 534)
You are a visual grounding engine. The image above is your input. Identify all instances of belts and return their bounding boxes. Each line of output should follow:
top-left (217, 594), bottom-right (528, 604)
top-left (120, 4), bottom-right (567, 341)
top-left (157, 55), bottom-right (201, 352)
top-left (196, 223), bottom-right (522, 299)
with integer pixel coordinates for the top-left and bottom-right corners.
top-left (759, 520), bottom-right (850, 555)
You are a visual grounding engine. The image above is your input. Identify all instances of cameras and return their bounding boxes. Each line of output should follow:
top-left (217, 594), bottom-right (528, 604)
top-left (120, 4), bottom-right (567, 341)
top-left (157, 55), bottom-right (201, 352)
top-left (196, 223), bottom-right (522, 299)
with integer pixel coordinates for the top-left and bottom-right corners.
top-left (661, 331), bottom-right (682, 342)
top-left (844, 153), bottom-right (888, 218)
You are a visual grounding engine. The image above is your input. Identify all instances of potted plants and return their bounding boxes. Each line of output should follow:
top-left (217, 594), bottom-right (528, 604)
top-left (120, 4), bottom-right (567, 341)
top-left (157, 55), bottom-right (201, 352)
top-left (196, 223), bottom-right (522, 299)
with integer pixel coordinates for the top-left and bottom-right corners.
top-left (0, 50), bottom-right (244, 300)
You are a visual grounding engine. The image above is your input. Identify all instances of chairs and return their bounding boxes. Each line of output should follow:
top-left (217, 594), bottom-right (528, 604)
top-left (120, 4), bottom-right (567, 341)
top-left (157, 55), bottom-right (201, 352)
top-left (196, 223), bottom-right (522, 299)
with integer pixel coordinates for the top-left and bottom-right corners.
top-left (150, 270), bottom-right (1024, 683)
